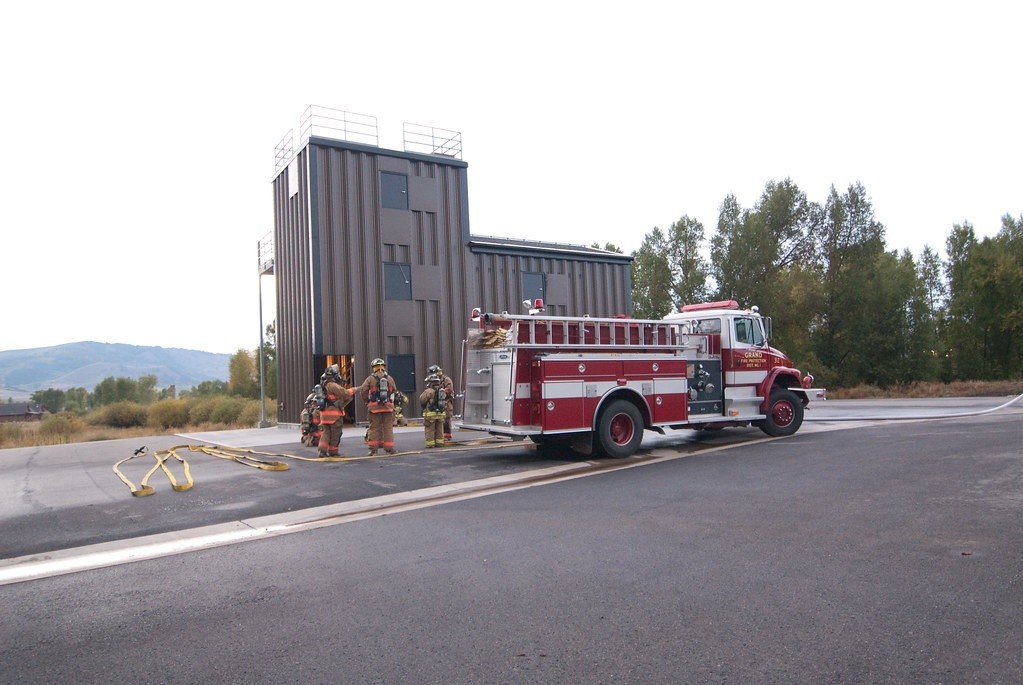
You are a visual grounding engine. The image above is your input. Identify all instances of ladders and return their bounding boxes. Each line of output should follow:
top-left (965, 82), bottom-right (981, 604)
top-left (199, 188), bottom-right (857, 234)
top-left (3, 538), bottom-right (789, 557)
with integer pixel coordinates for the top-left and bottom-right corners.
top-left (502, 315), bottom-right (690, 351)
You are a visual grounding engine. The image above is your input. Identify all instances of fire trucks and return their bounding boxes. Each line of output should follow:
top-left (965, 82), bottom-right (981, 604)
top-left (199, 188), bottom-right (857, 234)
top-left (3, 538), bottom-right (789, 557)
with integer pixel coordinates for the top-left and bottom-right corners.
top-left (453, 298), bottom-right (828, 460)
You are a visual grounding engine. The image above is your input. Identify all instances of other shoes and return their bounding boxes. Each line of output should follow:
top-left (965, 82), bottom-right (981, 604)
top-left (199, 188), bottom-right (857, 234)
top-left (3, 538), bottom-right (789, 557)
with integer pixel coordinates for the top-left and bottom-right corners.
top-left (368, 449), bottom-right (377, 456)
top-left (318, 452), bottom-right (328, 458)
top-left (330, 453), bottom-right (345, 458)
top-left (385, 449), bottom-right (398, 455)
top-left (426, 445), bottom-right (434, 448)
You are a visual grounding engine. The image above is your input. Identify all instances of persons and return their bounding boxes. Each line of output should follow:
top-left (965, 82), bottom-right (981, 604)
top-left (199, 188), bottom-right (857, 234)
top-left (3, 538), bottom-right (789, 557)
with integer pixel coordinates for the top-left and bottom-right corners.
top-left (393, 390), bottom-right (409, 426)
top-left (300, 364), bottom-right (363, 457)
top-left (419, 365), bottom-right (455, 447)
top-left (361, 358), bottom-right (399, 456)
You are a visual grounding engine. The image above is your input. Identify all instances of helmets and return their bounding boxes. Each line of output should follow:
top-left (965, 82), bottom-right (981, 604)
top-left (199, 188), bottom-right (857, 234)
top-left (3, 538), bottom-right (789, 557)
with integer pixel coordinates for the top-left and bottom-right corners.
top-left (371, 358), bottom-right (386, 366)
top-left (424, 374), bottom-right (440, 381)
top-left (428, 365), bottom-right (442, 373)
top-left (325, 367), bottom-right (338, 375)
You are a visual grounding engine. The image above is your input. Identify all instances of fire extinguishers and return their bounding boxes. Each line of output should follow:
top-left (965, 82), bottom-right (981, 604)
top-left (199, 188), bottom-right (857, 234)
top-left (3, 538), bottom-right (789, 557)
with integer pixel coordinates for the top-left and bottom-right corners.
top-left (302, 411), bottom-right (310, 435)
top-left (380, 377), bottom-right (388, 402)
top-left (314, 384), bottom-right (326, 411)
top-left (438, 388), bottom-right (445, 414)
